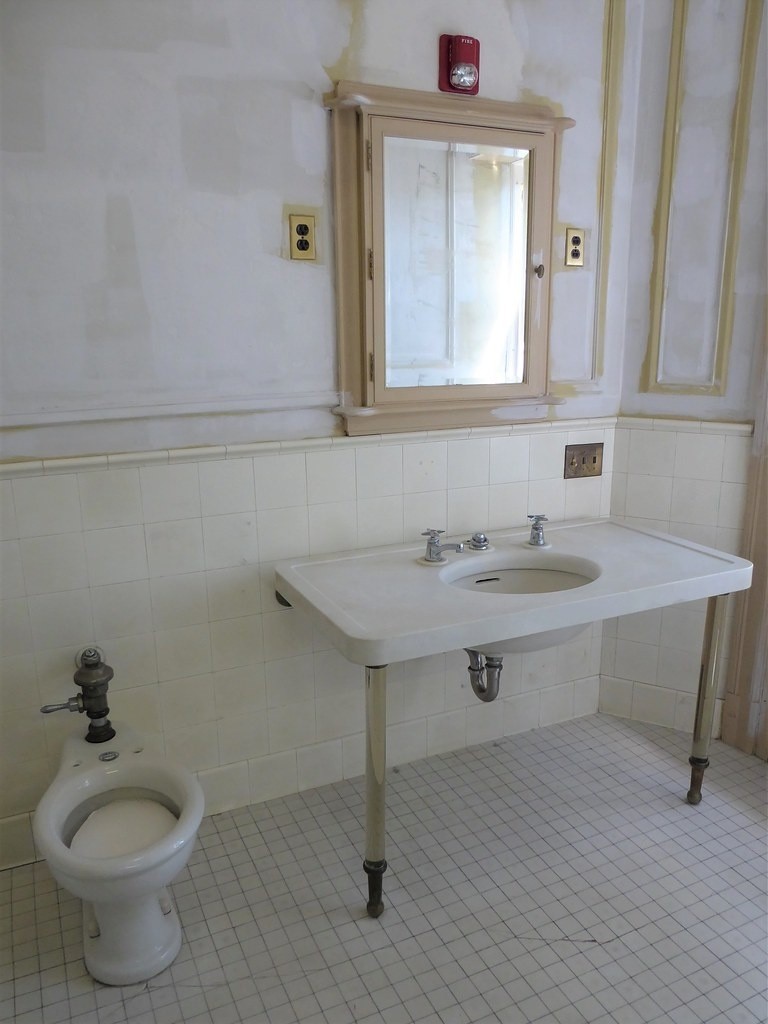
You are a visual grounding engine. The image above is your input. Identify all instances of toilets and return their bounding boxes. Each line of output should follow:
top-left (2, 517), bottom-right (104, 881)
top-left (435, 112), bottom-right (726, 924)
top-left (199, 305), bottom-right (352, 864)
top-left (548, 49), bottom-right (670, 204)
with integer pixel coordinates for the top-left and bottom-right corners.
top-left (29, 645), bottom-right (208, 990)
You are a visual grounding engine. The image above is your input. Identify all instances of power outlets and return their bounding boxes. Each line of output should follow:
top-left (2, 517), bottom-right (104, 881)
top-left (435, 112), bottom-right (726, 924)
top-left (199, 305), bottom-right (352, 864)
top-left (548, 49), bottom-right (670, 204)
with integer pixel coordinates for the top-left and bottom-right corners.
top-left (289, 214), bottom-right (315, 259)
top-left (565, 228), bottom-right (585, 267)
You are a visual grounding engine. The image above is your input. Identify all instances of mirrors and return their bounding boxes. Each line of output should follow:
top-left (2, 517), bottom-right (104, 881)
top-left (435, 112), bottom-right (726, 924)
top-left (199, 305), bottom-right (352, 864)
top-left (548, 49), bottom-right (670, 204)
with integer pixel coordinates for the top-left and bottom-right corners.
top-left (359, 103), bottom-right (548, 407)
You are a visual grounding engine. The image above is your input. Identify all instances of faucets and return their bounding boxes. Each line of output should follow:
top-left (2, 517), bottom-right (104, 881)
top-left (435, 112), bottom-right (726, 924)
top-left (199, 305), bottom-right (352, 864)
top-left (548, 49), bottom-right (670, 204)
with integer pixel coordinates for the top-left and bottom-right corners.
top-left (525, 513), bottom-right (549, 546)
top-left (419, 527), bottom-right (465, 563)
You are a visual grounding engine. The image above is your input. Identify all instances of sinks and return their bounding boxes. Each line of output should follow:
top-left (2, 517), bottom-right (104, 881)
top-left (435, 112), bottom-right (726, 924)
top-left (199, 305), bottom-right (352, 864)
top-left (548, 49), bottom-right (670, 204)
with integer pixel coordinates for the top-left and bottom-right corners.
top-left (438, 550), bottom-right (604, 596)
